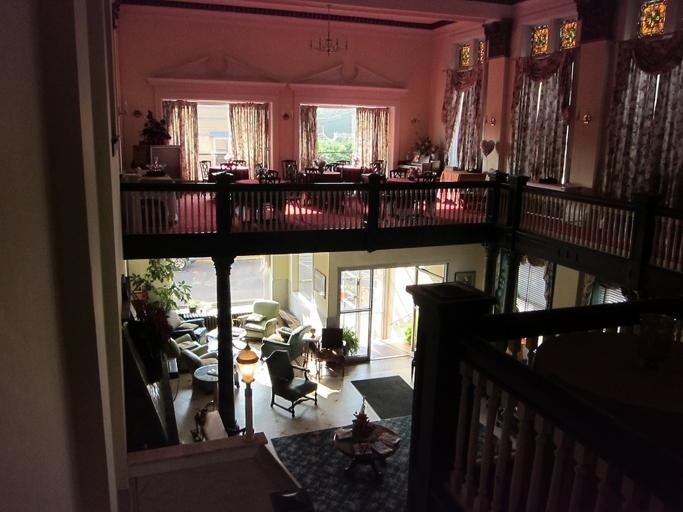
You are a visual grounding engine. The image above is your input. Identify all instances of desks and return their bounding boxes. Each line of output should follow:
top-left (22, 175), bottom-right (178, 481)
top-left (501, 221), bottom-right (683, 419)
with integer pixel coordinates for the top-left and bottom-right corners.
top-left (123, 169), bottom-right (179, 225)
top-left (191, 364), bottom-right (217, 411)
top-left (439, 169), bottom-right (484, 203)
top-left (333, 422), bottom-right (399, 479)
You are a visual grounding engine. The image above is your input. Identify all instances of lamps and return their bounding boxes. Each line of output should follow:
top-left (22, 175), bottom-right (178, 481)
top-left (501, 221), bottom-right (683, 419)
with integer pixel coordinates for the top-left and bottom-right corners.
top-left (236, 342), bottom-right (258, 438)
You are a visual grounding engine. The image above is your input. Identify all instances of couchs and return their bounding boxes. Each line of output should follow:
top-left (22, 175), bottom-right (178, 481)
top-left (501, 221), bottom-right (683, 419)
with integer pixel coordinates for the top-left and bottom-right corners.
top-left (236, 299), bottom-right (279, 342)
top-left (165, 311), bottom-right (209, 344)
top-left (182, 350), bottom-right (218, 398)
top-left (260, 325), bottom-right (311, 362)
top-left (167, 333), bottom-right (208, 370)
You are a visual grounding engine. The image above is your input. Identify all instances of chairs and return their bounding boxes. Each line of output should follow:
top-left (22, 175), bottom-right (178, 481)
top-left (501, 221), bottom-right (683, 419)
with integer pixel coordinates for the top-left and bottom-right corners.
top-left (266, 350), bottom-right (317, 416)
top-left (198, 155), bottom-right (438, 229)
top-left (199, 410), bottom-right (253, 442)
top-left (317, 328), bottom-right (345, 378)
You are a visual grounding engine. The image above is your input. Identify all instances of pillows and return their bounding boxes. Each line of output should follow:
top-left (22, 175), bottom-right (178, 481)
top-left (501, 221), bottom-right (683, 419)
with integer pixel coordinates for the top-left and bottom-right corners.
top-left (177, 323), bottom-right (198, 330)
top-left (246, 313), bottom-right (265, 322)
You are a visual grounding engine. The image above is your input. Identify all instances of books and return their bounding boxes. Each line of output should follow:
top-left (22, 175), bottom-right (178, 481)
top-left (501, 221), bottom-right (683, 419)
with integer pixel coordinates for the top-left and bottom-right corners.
top-left (351, 429), bottom-right (403, 457)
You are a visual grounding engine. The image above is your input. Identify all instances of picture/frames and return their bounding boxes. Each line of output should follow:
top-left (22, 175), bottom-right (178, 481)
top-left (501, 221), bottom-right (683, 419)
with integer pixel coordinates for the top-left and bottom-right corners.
top-left (313, 268), bottom-right (325, 298)
top-left (454, 271), bottom-right (476, 289)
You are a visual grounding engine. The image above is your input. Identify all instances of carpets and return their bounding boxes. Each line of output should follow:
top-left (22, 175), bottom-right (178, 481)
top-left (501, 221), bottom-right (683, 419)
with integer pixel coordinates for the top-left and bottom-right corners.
top-left (270, 415), bottom-right (485, 512)
top-left (350, 375), bottom-right (412, 419)
